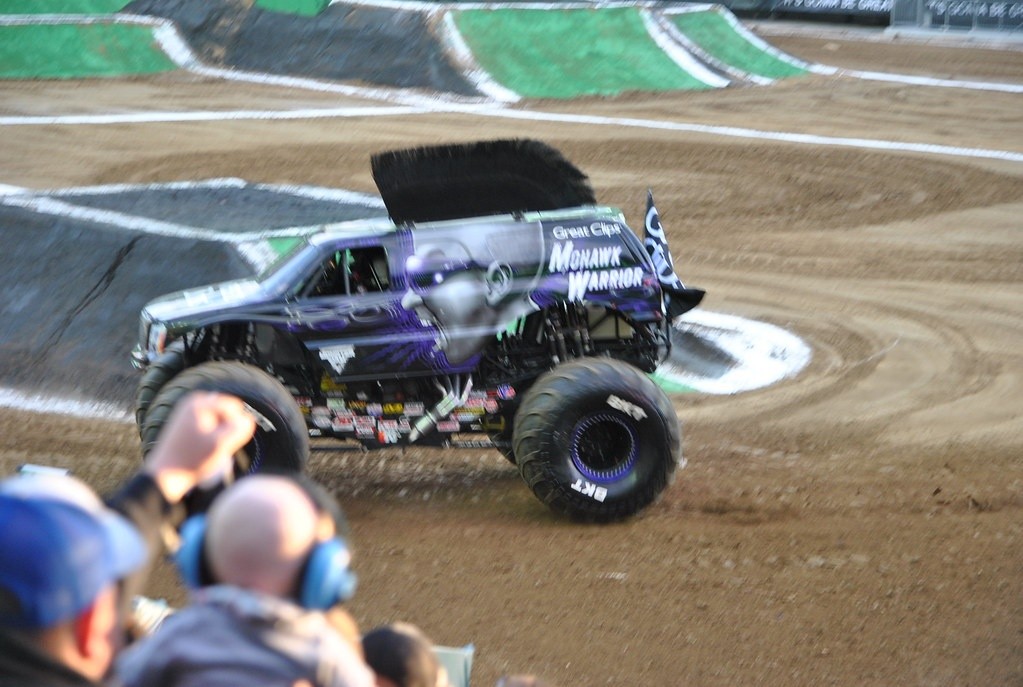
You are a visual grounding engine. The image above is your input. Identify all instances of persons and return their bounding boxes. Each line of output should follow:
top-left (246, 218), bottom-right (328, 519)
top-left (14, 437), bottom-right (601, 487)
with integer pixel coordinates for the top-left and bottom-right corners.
top-left (0, 390), bottom-right (461, 686)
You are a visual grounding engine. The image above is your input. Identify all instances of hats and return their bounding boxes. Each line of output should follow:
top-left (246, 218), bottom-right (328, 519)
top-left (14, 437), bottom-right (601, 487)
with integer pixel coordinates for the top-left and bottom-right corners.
top-left (0, 473), bottom-right (146, 627)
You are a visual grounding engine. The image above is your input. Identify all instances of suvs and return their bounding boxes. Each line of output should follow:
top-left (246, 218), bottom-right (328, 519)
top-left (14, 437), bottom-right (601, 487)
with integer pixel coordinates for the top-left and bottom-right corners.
top-left (128, 139), bottom-right (706, 527)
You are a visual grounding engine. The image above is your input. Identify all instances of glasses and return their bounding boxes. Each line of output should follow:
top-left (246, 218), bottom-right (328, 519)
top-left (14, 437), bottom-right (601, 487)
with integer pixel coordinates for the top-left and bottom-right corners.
top-left (405, 256), bottom-right (490, 288)
